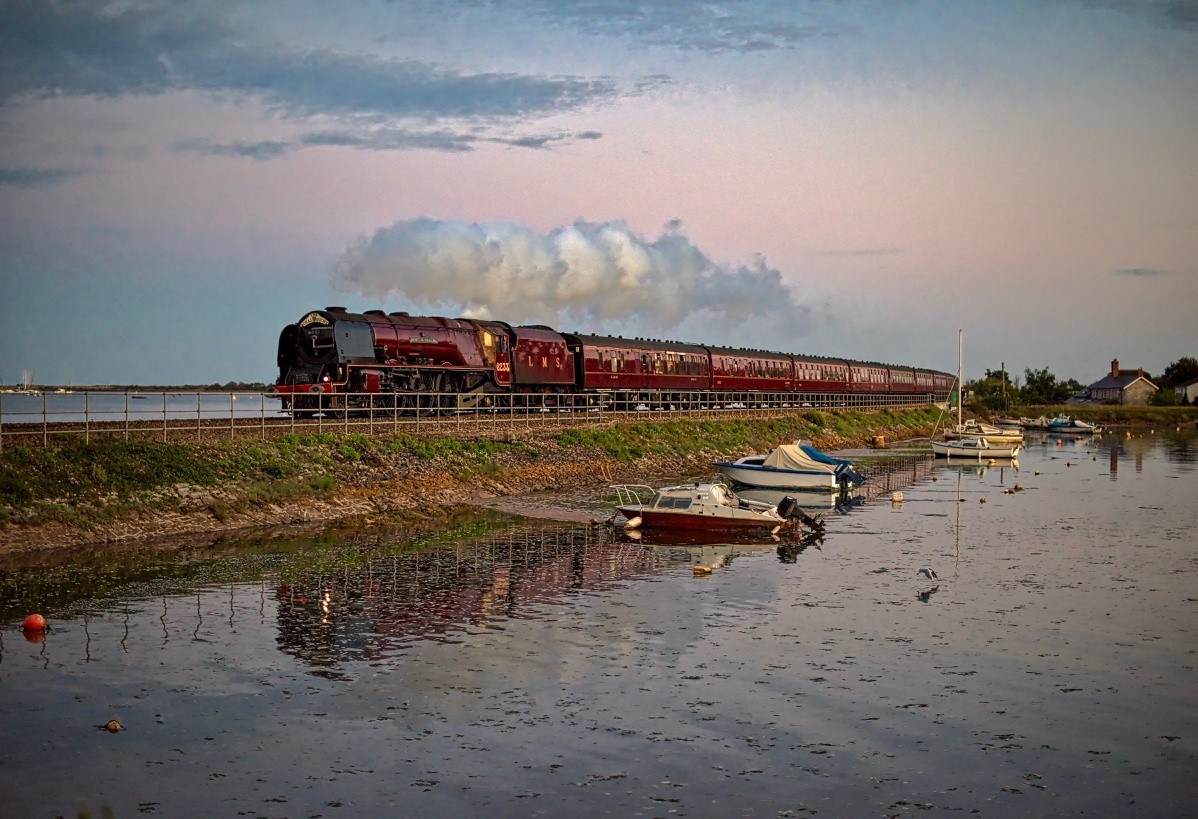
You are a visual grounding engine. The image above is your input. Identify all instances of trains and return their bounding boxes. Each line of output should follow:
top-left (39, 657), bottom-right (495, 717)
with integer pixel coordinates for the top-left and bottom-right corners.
top-left (263, 305), bottom-right (960, 421)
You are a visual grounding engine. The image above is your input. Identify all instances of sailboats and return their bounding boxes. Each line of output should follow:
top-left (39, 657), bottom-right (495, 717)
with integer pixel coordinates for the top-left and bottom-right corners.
top-left (931, 329), bottom-right (1021, 460)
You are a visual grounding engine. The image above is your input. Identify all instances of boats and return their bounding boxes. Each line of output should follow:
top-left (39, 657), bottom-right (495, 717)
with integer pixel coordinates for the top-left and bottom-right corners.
top-left (711, 438), bottom-right (868, 492)
top-left (607, 480), bottom-right (829, 534)
top-left (942, 410), bottom-right (1108, 442)
